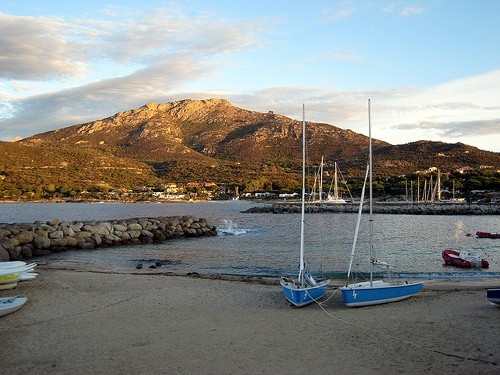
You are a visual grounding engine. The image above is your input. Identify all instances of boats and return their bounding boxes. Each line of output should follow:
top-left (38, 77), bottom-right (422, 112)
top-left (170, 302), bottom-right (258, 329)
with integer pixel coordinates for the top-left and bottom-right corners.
top-left (0, 296), bottom-right (28, 316)
top-left (442, 249), bottom-right (489, 269)
top-left (0, 260), bottom-right (38, 291)
top-left (475, 231), bottom-right (500, 239)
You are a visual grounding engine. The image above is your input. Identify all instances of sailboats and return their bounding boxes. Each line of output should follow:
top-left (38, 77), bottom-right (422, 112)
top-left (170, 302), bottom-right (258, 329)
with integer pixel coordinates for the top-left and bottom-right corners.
top-left (338, 97), bottom-right (426, 306)
top-left (322, 161), bottom-right (355, 203)
top-left (305, 155), bottom-right (324, 204)
top-left (373, 166), bottom-right (468, 207)
top-left (281, 104), bottom-right (330, 307)
